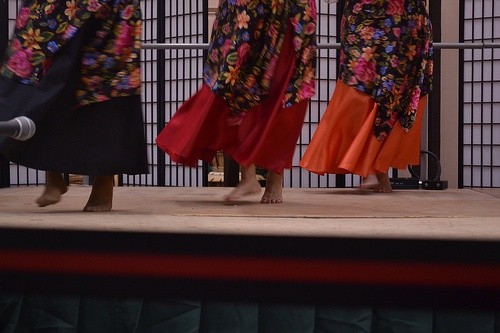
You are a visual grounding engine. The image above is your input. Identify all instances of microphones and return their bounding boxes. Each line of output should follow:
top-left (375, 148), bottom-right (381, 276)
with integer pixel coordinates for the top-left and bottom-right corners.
top-left (0, 116), bottom-right (35, 141)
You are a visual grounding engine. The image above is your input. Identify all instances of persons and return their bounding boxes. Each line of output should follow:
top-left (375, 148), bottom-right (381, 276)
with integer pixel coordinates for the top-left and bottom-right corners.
top-left (0, 0), bottom-right (152, 212)
top-left (154, 0), bottom-right (317, 204)
top-left (298, 0), bottom-right (434, 193)
